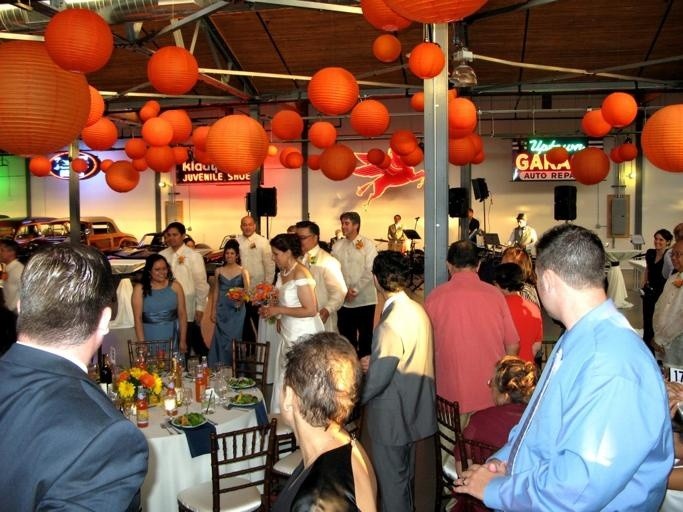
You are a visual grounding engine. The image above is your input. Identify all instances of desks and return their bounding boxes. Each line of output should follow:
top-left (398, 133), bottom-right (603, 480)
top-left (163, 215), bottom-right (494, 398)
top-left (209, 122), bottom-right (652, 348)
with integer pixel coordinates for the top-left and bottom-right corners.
top-left (602, 246), bottom-right (644, 310)
top-left (106, 260), bottom-right (148, 328)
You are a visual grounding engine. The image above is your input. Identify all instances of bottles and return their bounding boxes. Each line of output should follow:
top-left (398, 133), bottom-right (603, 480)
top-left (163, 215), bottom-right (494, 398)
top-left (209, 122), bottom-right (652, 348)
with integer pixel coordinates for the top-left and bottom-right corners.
top-left (135, 349), bottom-right (167, 378)
top-left (100, 352), bottom-right (112, 395)
top-left (166, 375), bottom-right (177, 416)
top-left (136, 385), bottom-right (149, 427)
top-left (169, 350), bottom-right (208, 401)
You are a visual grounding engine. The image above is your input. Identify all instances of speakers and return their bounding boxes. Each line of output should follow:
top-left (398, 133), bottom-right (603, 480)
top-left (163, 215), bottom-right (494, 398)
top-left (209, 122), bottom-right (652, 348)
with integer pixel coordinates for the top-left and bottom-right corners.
top-left (471, 177), bottom-right (489, 200)
top-left (553, 185), bottom-right (577, 220)
top-left (256, 188), bottom-right (277, 216)
top-left (449, 188), bottom-right (469, 217)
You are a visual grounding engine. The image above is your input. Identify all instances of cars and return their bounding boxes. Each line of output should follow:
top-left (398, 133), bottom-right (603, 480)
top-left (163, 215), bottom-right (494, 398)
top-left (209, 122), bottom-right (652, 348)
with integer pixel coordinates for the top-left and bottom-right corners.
top-left (0, 216), bottom-right (242, 279)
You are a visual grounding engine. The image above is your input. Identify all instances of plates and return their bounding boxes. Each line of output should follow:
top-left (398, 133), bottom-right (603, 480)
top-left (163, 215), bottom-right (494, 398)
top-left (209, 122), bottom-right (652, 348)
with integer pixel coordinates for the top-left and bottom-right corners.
top-left (173, 412), bottom-right (207, 428)
top-left (232, 401), bottom-right (256, 406)
top-left (227, 382), bottom-right (257, 389)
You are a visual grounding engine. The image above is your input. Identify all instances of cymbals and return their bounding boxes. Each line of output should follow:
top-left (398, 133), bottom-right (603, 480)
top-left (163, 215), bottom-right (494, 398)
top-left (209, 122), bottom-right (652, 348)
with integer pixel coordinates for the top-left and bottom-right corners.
top-left (375, 239), bottom-right (388, 242)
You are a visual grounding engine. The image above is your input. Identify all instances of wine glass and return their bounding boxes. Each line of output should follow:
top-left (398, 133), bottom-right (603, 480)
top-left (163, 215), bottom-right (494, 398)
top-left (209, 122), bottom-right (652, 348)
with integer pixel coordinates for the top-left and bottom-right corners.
top-left (180, 387), bottom-right (192, 413)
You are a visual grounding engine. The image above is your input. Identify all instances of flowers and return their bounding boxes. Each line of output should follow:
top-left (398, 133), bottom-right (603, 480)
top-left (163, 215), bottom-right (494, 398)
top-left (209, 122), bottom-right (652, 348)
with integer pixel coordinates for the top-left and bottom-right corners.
top-left (225, 283), bottom-right (280, 319)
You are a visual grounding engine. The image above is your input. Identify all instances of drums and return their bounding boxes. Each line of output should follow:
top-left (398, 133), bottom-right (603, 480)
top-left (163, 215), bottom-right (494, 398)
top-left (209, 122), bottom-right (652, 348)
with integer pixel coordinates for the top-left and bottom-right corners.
top-left (388, 240), bottom-right (404, 253)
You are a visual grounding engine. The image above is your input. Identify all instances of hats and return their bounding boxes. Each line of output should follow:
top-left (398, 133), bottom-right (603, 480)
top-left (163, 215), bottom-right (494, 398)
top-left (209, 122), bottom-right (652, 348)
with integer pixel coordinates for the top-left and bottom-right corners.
top-left (516, 213), bottom-right (528, 221)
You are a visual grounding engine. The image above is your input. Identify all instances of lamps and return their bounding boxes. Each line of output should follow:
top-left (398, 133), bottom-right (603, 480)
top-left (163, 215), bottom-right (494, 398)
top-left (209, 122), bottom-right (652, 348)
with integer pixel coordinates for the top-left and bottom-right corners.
top-left (447, 46), bottom-right (481, 91)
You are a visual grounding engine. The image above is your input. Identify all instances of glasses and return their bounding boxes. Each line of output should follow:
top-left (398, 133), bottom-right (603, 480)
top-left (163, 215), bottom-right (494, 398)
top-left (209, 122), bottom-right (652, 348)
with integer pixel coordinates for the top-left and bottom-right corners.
top-left (668, 251), bottom-right (683, 258)
top-left (298, 234), bottom-right (312, 241)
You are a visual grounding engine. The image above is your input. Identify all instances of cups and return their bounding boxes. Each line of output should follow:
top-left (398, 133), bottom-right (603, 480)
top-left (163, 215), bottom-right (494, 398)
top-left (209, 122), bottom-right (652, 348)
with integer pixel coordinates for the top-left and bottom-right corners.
top-left (214, 360), bottom-right (233, 398)
top-left (200, 393), bottom-right (215, 413)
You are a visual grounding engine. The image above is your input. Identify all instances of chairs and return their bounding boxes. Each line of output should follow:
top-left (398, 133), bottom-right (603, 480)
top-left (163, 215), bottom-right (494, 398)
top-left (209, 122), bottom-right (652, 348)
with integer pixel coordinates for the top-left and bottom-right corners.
top-left (95, 338), bottom-right (306, 512)
top-left (433, 395), bottom-right (502, 512)
